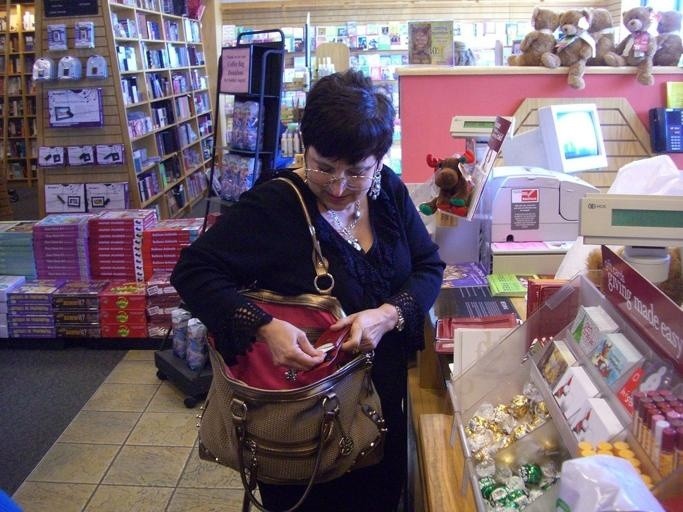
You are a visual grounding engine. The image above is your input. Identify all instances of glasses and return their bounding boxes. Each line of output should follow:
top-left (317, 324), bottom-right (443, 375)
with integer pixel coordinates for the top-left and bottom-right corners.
top-left (301, 148), bottom-right (381, 194)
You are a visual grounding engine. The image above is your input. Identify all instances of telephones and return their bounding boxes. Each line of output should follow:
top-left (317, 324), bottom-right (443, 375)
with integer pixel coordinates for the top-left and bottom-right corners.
top-left (649, 107), bottom-right (683, 153)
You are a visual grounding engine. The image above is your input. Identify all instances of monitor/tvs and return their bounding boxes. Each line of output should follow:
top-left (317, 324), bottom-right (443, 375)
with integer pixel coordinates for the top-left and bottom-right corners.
top-left (503, 104), bottom-right (608, 174)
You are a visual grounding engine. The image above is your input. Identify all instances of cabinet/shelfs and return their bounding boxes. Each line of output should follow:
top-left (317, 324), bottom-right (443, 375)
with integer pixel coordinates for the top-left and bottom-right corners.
top-left (0, 0), bottom-right (285, 223)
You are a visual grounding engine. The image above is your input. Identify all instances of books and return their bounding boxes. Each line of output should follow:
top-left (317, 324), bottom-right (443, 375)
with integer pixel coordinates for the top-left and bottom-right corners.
top-left (136, 0), bottom-right (213, 215)
top-left (0, 11), bottom-right (37, 182)
top-left (431, 259), bottom-right (581, 360)
top-left (109, 0), bottom-right (159, 202)
top-left (281, 26), bottom-right (403, 159)
top-left (0, 208), bottom-right (220, 342)
top-left (408, 20), bottom-right (454, 64)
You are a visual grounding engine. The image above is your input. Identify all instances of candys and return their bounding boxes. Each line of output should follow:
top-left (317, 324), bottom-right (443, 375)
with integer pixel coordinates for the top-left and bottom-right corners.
top-left (466, 395), bottom-right (560, 512)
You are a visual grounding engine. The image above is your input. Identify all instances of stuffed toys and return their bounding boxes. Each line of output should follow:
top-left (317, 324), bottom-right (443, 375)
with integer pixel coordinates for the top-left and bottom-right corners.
top-left (509, 4), bottom-right (683, 90)
top-left (423, 154), bottom-right (473, 216)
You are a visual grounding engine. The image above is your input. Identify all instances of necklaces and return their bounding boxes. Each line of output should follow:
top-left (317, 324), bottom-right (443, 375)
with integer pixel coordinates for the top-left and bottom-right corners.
top-left (321, 201), bottom-right (361, 245)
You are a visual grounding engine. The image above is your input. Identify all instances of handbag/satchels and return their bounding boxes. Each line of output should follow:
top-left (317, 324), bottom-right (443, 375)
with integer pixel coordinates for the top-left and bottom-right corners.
top-left (192, 173), bottom-right (390, 512)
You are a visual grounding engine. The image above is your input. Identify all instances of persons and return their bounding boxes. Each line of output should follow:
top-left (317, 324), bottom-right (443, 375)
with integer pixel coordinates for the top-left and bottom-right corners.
top-left (169, 74), bottom-right (444, 512)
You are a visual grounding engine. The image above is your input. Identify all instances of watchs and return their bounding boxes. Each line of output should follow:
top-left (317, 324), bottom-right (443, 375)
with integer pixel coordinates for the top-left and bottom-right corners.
top-left (396, 305), bottom-right (405, 332)
